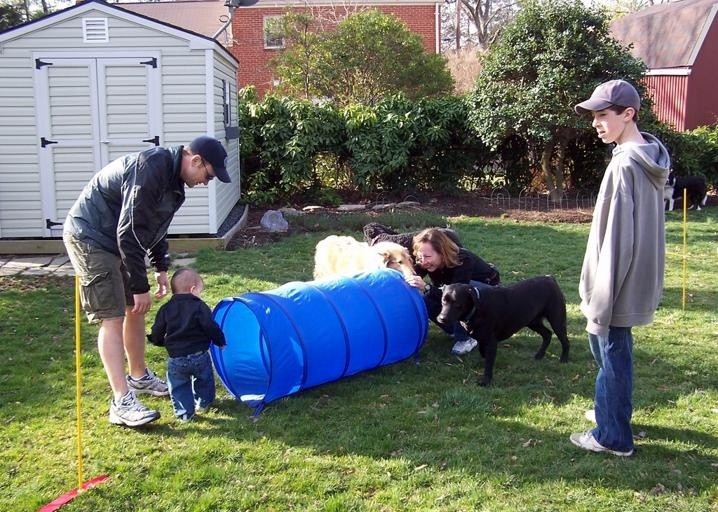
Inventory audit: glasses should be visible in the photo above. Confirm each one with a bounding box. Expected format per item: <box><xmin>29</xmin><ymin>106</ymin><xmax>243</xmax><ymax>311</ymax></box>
<box><xmin>201</xmin><ymin>159</ymin><xmax>214</xmax><ymax>181</ymax></box>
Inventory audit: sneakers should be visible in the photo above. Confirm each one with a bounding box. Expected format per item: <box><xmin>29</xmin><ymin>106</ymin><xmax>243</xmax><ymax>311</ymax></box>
<box><xmin>125</xmin><ymin>367</ymin><xmax>168</xmax><ymax>397</ymax></box>
<box><xmin>586</xmin><ymin>409</ymin><xmax>597</xmax><ymax>424</ymax></box>
<box><xmin>569</xmin><ymin>431</ymin><xmax>633</xmax><ymax>457</ymax></box>
<box><xmin>178</xmin><ymin>400</ymin><xmax>210</xmax><ymax>423</ymax></box>
<box><xmin>108</xmin><ymin>390</ymin><xmax>161</xmax><ymax>428</ymax></box>
<box><xmin>452</xmin><ymin>338</ymin><xmax>477</xmax><ymax>356</ymax></box>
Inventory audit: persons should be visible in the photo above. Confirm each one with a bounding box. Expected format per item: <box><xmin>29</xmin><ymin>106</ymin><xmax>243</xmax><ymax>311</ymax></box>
<box><xmin>61</xmin><ymin>136</ymin><xmax>231</xmax><ymax>428</ymax></box>
<box><xmin>412</xmin><ymin>229</ymin><xmax>501</xmax><ymax>356</ymax></box>
<box><xmin>146</xmin><ymin>267</ymin><xmax>226</xmax><ymax>422</ymax></box>
<box><xmin>568</xmin><ymin>80</ymin><xmax>670</xmax><ymax>459</ymax></box>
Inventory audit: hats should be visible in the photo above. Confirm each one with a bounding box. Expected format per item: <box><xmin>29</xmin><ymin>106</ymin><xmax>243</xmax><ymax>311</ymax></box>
<box><xmin>190</xmin><ymin>135</ymin><xmax>232</xmax><ymax>184</ymax></box>
<box><xmin>575</xmin><ymin>78</ymin><xmax>641</xmax><ymax>114</ymax></box>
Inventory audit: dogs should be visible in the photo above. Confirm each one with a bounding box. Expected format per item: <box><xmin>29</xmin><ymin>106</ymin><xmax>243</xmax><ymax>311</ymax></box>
<box><xmin>362</xmin><ymin>222</ymin><xmax>463</xmax><ymax>280</ymax></box>
<box><xmin>312</xmin><ymin>233</ymin><xmax>418</xmax><ymax>284</ymax></box>
<box><xmin>436</xmin><ymin>273</ymin><xmax>571</xmax><ymax>388</ymax></box>
